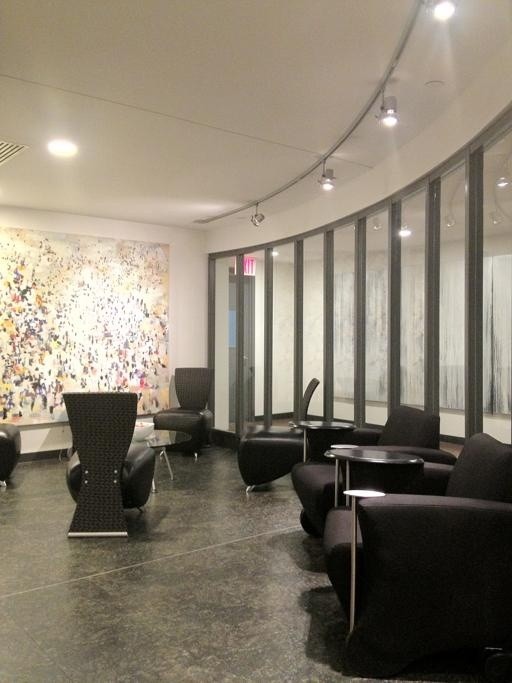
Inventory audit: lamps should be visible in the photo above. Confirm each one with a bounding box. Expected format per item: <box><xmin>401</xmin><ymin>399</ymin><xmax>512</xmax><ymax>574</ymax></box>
<box><xmin>425</xmin><ymin>1</ymin><xmax>456</xmax><ymax>20</ymax></box>
<box><xmin>380</xmin><ymin>88</ymin><xmax>398</xmax><ymax>127</ymax></box>
<box><xmin>399</xmin><ymin>224</ymin><xmax>411</xmax><ymax>237</ymax></box>
<box><xmin>444</xmin><ymin>212</ymin><xmax>456</xmax><ymax>227</ymax></box>
<box><xmin>489</xmin><ymin>211</ymin><xmax>503</xmax><ymax>225</ymax></box>
<box><xmin>272</xmin><ymin>249</ymin><xmax>280</xmax><ymax>258</ymax></box>
<box><xmin>322</xmin><ymin>160</ymin><xmax>334</xmax><ymax>191</ymax></box>
<box><xmin>371</xmin><ymin>218</ymin><xmax>383</xmax><ymax>231</ymax></box>
<box><xmin>251</xmin><ymin>204</ymin><xmax>265</xmax><ymax>226</ymax></box>
<box><xmin>496</xmin><ymin>176</ymin><xmax>511</xmax><ymax>189</ymax></box>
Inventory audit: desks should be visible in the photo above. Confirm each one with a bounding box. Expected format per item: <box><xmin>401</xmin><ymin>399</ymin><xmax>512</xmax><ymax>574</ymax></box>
<box><xmin>290</xmin><ymin>421</ymin><xmax>354</xmax><ymax>463</ymax></box>
<box><xmin>324</xmin><ymin>448</ymin><xmax>424</xmax><ymax>507</ymax></box>
<box><xmin>130</xmin><ymin>423</ymin><xmax>192</xmax><ymax>482</ymax></box>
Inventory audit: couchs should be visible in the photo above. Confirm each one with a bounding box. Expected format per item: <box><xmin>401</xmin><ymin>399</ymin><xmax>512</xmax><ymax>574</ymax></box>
<box><xmin>292</xmin><ymin>405</ymin><xmax>464</xmax><ymax>532</ymax></box>
<box><xmin>324</xmin><ymin>432</ymin><xmax>512</xmax><ymax>679</ymax></box>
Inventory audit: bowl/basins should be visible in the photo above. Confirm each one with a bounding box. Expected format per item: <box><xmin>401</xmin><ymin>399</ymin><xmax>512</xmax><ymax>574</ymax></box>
<box><xmin>132</xmin><ymin>422</ymin><xmax>155</xmax><ymax>441</ymax></box>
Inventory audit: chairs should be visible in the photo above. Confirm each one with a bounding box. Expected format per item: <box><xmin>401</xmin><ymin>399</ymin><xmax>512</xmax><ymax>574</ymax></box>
<box><xmin>153</xmin><ymin>367</ymin><xmax>215</xmax><ymax>460</ymax></box>
<box><xmin>238</xmin><ymin>379</ymin><xmax>318</xmax><ymax>493</ymax></box>
<box><xmin>0</xmin><ymin>423</ymin><xmax>20</xmax><ymax>487</ymax></box>
<box><xmin>63</xmin><ymin>392</ymin><xmax>156</xmax><ymax>537</ymax></box>
